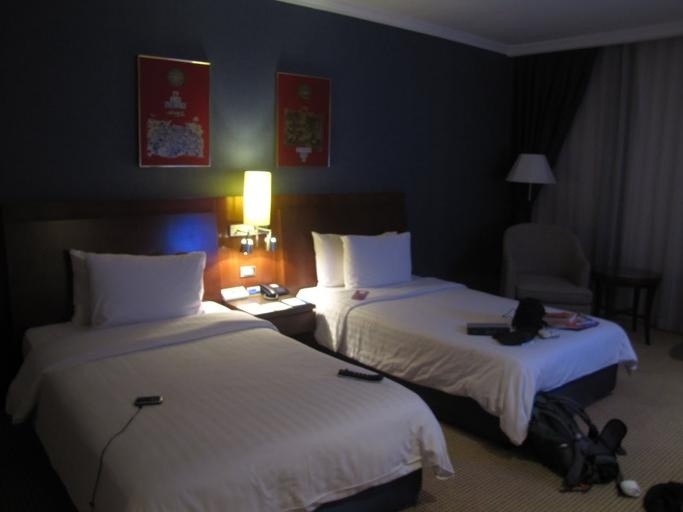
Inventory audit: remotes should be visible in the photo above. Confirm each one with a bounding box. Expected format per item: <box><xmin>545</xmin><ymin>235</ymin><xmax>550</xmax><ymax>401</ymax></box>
<box><xmin>337</xmin><ymin>366</ymin><xmax>384</xmax><ymax>383</ymax></box>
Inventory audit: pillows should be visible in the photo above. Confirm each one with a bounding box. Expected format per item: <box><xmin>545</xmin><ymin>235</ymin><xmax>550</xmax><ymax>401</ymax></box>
<box><xmin>68</xmin><ymin>248</ymin><xmax>92</xmax><ymax>326</ymax></box>
<box><xmin>85</xmin><ymin>251</ymin><xmax>207</xmax><ymax>329</ymax></box>
<box><xmin>339</xmin><ymin>231</ymin><xmax>412</xmax><ymax>289</ymax></box>
<box><xmin>311</xmin><ymin>230</ymin><xmax>398</xmax><ymax>288</ymax></box>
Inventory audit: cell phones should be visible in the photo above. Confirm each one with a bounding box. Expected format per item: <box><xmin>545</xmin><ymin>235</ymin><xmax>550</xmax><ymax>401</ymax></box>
<box><xmin>133</xmin><ymin>394</ymin><xmax>161</xmax><ymax>408</ymax></box>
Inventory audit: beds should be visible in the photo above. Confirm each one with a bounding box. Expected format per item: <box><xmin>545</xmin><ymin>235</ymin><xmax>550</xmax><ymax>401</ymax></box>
<box><xmin>1</xmin><ymin>197</ymin><xmax>456</xmax><ymax>512</ymax></box>
<box><xmin>277</xmin><ymin>193</ymin><xmax>639</xmax><ymax>449</ymax></box>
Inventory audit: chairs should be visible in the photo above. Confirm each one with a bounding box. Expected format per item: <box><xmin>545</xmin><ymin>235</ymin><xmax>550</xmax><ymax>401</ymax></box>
<box><xmin>500</xmin><ymin>222</ymin><xmax>594</xmax><ymax>315</ymax></box>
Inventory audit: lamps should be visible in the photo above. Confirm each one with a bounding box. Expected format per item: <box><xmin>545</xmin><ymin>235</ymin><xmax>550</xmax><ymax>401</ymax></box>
<box><xmin>229</xmin><ymin>170</ymin><xmax>277</xmax><ymax>255</ymax></box>
<box><xmin>504</xmin><ymin>153</ymin><xmax>557</xmax><ymax>202</ymax></box>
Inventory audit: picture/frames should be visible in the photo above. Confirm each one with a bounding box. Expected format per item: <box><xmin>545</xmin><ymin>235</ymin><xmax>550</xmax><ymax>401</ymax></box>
<box><xmin>137</xmin><ymin>54</ymin><xmax>211</xmax><ymax>169</ymax></box>
<box><xmin>276</xmin><ymin>71</ymin><xmax>332</xmax><ymax>169</ymax></box>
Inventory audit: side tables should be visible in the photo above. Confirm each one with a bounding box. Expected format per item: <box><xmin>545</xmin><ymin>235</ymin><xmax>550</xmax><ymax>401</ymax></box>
<box><xmin>596</xmin><ymin>264</ymin><xmax>662</xmax><ymax>345</ymax></box>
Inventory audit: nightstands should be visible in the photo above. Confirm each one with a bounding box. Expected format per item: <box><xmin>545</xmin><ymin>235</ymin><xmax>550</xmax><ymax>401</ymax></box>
<box><xmin>222</xmin><ymin>293</ymin><xmax>316</xmax><ymax>348</ymax></box>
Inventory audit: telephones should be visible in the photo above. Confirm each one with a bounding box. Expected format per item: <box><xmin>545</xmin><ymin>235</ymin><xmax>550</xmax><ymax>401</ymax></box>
<box><xmin>259</xmin><ymin>282</ymin><xmax>288</xmax><ymax>296</ymax></box>
